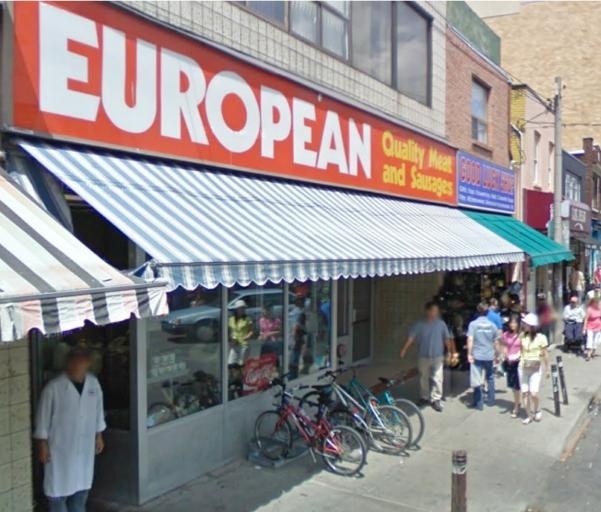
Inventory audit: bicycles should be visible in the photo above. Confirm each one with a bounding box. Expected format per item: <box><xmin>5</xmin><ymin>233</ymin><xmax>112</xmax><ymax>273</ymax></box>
<box><xmin>148</xmin><ymin>359</ymin><xmax>252</xmax><ymax>429</ymax></box>
<box><xmin>255</xmin><ymin>361</ymin><xmax>428</xmax><ymax>476</ymax></box>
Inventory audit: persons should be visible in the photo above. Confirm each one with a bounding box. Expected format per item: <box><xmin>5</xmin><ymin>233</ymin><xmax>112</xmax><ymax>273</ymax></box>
<box><xmin>399</xmin><ymin>264</ymin><xmax>601</xmax><ymax>425</ymax></box>
<box><xmin>227</xmin><ymin>291</ymin><xmax>331</xmax><ymax>382</ymax></box>
<box><xmin>32</xmin><ymin>346</ymin><xmax>108</xmax><ymax>512</ymax></box>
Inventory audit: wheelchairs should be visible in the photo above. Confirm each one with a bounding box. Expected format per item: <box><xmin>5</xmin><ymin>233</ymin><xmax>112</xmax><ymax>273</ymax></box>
<box><xmin>562</xmin><ymin>323</ymin><xmax>586</xmax><ymax>350</ymax></box>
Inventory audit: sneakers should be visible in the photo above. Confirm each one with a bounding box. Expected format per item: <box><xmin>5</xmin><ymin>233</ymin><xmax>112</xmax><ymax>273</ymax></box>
<box><xmin>433</xmin><ymin>400</ymin><xmax>443</xmax><ymax>411</ymax></box>
<box><xmin>416</xmin><ymin>398</ymin><xmax>429</xmax><ymax>408</ymax></box>
<box><xmin>536</xmin><ymin>410</ymin><xmax>543</xmax><ymax>421</ymax></box>
<box><xmin>511</xmin><ymin>408</ymin><xmax>520</xmax><ymax>418</ymax></box>
<box><xmin>523</xmin><ymin>414</ymin><xmax>534</xmax><ymax>424</ymax></box>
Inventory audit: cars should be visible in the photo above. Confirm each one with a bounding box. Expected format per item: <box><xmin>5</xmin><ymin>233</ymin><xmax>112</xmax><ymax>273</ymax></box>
<box><xmin>161</xmin><ymin>288</ymin><xmax>311</xmax><ymax>343</ymax></box>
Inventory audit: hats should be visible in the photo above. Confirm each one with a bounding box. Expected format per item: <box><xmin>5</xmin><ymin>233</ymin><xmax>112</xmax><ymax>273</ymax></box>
<box><xmin>570</xmin><ymin>296</ymin><xmax>578</xmax><ymax>303</ymax></box>
<box><xmin>523</xmin><ymin>313</ymin><xmax>540</xmax><ymax>327</ymax></box>
<box><xmin>228</xmin><ymin>299</ymin><xmax>249</xmax><ymax>310</ymax></box>
